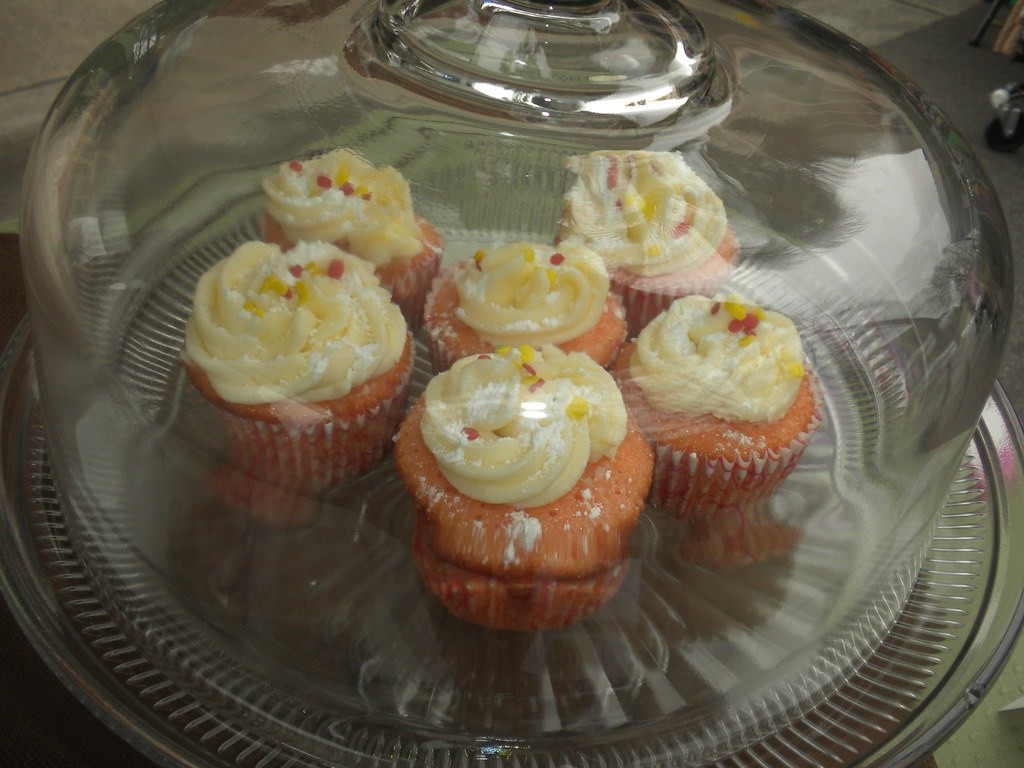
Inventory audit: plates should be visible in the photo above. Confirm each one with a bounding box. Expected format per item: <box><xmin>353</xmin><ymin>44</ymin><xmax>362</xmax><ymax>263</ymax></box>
<box><xmin>0</xmin><ymin>298</ymin><xmax>1024</xmax><ymax>768</ymax></box>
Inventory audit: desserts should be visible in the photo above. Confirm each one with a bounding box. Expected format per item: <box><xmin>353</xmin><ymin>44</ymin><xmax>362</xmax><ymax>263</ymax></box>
<box><xmin>179</xmin><ymin>148</ymin><xmax>824</xmax><ymax>631</ymax></box>
